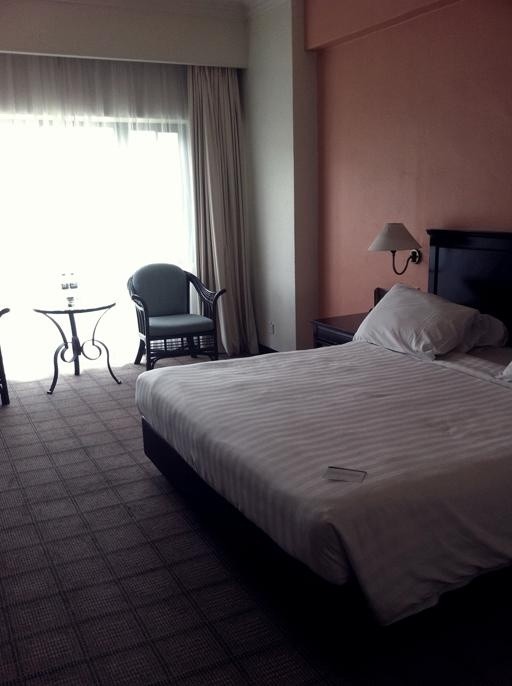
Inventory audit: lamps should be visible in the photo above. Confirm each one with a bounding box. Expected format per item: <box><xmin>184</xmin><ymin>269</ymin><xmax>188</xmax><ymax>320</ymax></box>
<box><xmin>367</xmin><ymin>222</ymin><xmax>423</xmax><ymax>275</ymax></box>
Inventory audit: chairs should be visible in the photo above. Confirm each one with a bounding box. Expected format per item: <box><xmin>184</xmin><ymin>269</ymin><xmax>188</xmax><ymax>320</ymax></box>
<box><xmin>127</xmin><ymin>264</ymin><xmax>227</xmax><ymax>370</ymax></box>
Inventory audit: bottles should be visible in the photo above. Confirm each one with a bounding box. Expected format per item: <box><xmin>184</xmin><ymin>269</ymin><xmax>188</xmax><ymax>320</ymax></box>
<box><xmin>61</xmin><ymin>272</ymin><xmax>78</xmax><ymax>303</ymax></box>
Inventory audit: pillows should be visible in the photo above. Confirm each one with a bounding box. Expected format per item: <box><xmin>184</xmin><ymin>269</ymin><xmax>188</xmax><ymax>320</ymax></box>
<box><xmin>352</xmin><ymin>283</ymin><xmax>512</xmax><ymax>381</ymax></box>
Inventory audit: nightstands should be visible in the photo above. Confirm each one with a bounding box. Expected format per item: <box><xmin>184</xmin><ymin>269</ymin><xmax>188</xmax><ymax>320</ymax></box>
<box><xmin>310</xmin><ymin>288</ymin><xmax>388</xmax><ymax>348</ymax></box>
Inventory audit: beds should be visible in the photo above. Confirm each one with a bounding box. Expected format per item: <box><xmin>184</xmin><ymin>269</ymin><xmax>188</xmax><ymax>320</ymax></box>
<box><xmin>142</xmin><ymin>228</ymin><xmax>512</xmax><ymax>676</ymax></box>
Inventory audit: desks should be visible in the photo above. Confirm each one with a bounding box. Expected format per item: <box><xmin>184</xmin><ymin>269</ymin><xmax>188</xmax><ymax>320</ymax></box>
<box><xmin>33</xmin><ymin>303</ymin><xmax>122</xmax><ymax>394</ymax></box>
